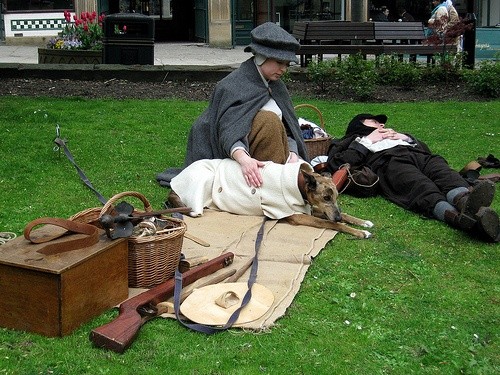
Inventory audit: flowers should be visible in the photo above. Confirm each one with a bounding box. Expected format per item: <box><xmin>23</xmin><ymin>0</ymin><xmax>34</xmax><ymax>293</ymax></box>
<box><xmin>48</xmin><ymin>10</ymin><xmax>126</xmax><ymax>49</ymax></box>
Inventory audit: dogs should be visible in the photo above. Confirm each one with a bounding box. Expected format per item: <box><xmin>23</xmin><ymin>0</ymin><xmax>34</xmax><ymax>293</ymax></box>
<box><xmin>166</xmin><ymin>164</ymin><xmax>375</xmax><ymax>240</ymax></box>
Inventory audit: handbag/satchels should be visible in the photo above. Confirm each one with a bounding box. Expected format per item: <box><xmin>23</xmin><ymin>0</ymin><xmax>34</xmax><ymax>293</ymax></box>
<box><xmin>339</xmin><ymin>163</ymin><xmax>379</xmax><ymax>198</ymax></box>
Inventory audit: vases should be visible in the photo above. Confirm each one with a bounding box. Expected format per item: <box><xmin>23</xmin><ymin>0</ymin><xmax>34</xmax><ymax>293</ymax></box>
<box><xmin>39</xmin><ymin>48</ymin><xmax>102</xmax><ymax>65</ymax></box>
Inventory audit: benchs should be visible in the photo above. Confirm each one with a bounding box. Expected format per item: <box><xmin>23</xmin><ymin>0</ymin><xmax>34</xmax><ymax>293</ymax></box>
<box><xmin>293</xmin><ymin>19</ymin><xmax>458</xmax><ymax>69</ymax></box>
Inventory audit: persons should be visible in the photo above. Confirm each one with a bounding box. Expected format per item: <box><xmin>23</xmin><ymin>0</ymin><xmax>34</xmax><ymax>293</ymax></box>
<box><xmin>423</xmin><ymin>0</ymin><xmax>463</xmax><ymax>46</ymax></box>
<box><xmin>185</xmin><ymin>22</ymin><xmax>311</xmax><ymax>188</ymax></box>
<box><xmin>371</xmin><ymin>7</ymin><xmax>416</xmax><ymax>22</ymax></box>
<box><xmin>328</xmin><ymin>114</ymin><xmax>500</xmax><ymax>242</ymax></box>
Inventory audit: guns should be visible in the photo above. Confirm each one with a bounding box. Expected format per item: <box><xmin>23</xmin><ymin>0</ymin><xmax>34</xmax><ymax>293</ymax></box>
<box><xmin>88</xmin><ymin>248</ymin><xmax>235</xmax><ymax>355</ymax></box>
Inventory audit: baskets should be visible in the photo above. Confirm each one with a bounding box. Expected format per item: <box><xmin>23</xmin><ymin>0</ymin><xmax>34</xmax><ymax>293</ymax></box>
<box><xmin>67</xmin><ymin>191</ymin><xmax>187</xmax><ymax>289</ymax></box>
<box><xmin>294</xmin><ymin>104</ymin><xmax>333</xmax><ymax>162</ymax></box>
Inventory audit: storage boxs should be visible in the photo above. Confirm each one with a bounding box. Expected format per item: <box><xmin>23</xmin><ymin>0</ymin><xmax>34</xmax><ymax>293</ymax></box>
<box><xmin>0</xmin><ymin>222</ymin><xmax>130</xmax><ymax>339</ymax></box>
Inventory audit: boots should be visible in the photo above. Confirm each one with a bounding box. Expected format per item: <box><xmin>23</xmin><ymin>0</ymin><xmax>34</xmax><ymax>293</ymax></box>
<box><xmin>444</xmin><ymin>206</ymin><xmax>500</xmax><ymax>243</ymax></box>
<box><xmin>453</xmin><ymin>181</ymin><xmax>496</xmax><ymax>229</ymax></box>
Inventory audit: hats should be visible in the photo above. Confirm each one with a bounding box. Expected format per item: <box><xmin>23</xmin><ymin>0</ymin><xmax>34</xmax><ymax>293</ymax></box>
<box><xmin>347</xmin><ymin>113</ymin><xmax>388</xmax><ymax>124</ymax></box>
<box><xmin>244</xmin><ymin>22</ymin><xmax>300</xmax><ymax>64</ymax></box>
